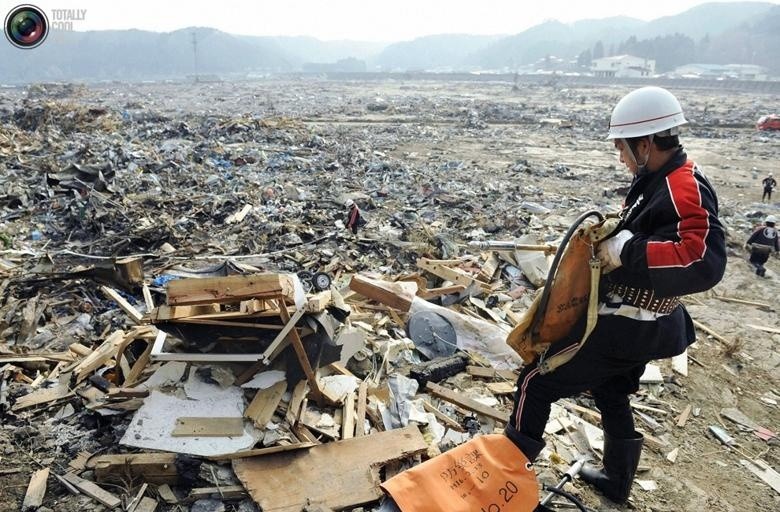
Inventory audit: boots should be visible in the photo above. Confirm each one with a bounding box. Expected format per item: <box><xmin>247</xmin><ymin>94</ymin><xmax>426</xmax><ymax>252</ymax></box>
<box><xmin>579</xmin><ymin>430</ymin><xmax>644</xmax><ymax>504</ymax></box>
<box><xmin>758</xmin><ymin>265</ymin><xmax>766</xmax><ymax>276</ymax></box>
<box><xmin>504</xmin><ymin>424</ymin><xmax>546</xmax><ymax>463</ymax></box>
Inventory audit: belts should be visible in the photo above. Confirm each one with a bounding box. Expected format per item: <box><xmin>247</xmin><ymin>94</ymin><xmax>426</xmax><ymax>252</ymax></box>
<box><xmin>614</xmin><ymin>284</ymin><xmax>679</xmax><ymax>314</ymax></box>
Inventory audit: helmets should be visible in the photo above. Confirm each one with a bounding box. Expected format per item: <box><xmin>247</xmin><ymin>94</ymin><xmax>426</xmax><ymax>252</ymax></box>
<box><xmin>765</xmin><ymin>215</ymin><xmax>776</xmax><ymax>224</ymax></box>
<box><xmin>604</xmin><ymin>85</ymin><xmax>688</xmax><ymax>141</ymax></box>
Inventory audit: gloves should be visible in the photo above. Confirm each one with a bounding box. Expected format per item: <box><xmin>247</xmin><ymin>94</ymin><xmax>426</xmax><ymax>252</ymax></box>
<box><xmin>596</xmin><ymin>230</ymin><xmax>632</xmax><ymax>276</ymax></box>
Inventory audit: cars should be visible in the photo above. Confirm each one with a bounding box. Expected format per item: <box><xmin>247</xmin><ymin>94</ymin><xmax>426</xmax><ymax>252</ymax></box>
<box><xmin>755</xmin><ymin>115</ymin><xmax>780</xmax><ymax>131</ymax></box>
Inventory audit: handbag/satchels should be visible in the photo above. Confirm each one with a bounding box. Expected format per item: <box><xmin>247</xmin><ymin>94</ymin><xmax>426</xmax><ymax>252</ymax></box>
<box><xmin>379</xmin><ymin>433</ymin><xmax>540</xmax><ymax>511</ymax></box>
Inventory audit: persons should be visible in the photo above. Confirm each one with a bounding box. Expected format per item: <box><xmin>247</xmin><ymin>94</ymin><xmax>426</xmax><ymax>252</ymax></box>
<box><xmin>744</xmin><ymin>214</ymin><xmax>780</xmax><ymax>277</ymax></box>
<box><xmin>503</xmin><ymin>85</ymin><xmax>728</xmax><ymax>505</ymax></box>
<box><xmin>762</xmin><ymin>173</ymin><xmax>777</xmax><ymax>203</ymax></box>
<box><xmin>345</xmin><ymin>199</ymin><xmax>361</xmax><ymax>236</ymax></box>
